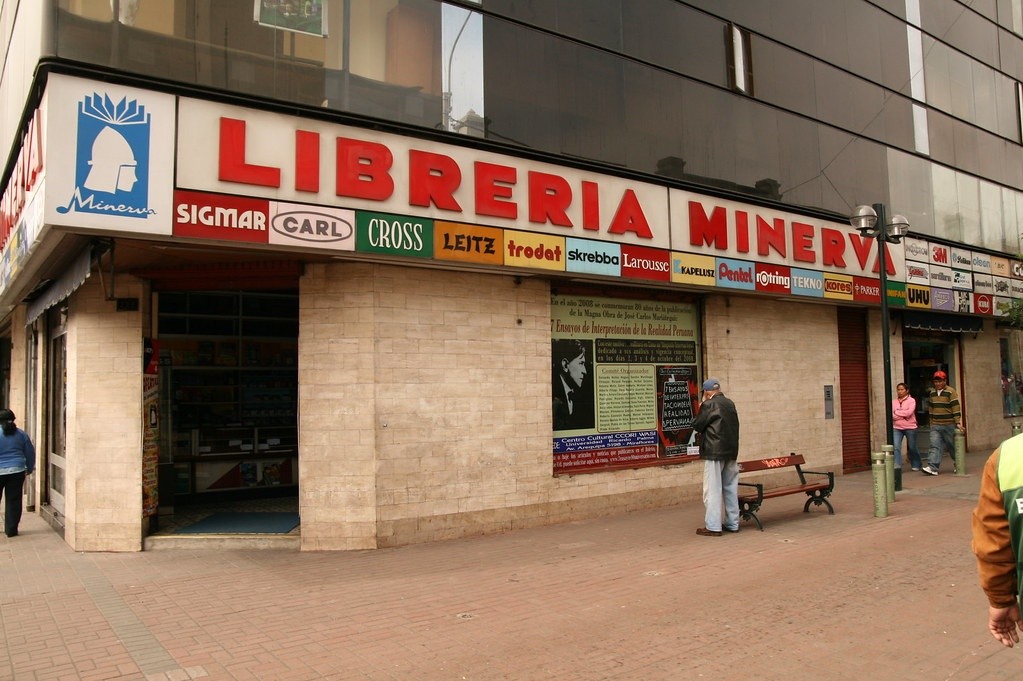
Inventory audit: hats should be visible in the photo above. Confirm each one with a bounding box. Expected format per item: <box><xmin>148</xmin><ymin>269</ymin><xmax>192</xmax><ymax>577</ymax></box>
<box><xmin>932</xmin><ymin>370</ymin><xmax>947</xmax><ymax>381</ymax></box>
<box><xmin>700</xmin><ymin>377</ymin><xmax>720</xmax><ymax>393</ymax></box>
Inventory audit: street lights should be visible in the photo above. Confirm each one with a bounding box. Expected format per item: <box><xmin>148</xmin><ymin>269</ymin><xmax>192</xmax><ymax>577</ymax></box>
<box><xmin>848</xmin><ymin>203</ymin><xmax>911</xmax><ymax>503</ymax></box>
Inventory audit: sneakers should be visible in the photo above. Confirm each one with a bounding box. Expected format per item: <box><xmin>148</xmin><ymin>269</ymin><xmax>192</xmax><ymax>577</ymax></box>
<box><xmin>923</xmin><ymin>466</ymin><xmax>939</xmax><ymax>476</ymax></box>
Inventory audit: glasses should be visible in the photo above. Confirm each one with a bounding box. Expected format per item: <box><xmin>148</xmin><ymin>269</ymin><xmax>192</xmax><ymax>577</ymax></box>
<box><xmin>933</xmin><ymin>377</ymin><xmax>942</xmax><ymax>380</ymax></box>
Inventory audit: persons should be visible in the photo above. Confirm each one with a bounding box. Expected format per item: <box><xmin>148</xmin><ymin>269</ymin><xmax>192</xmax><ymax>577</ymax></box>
<box><xmin>892</xmin><ymin>383</ymin><xmax>923</xmax><ymax>471</ymax></box>
<box><xmin>690</xmin><ymin>377</ymin><xmax>742</xmax><ymax>537</ymax></box>
<box><xmin>552</xmin><ymin>339</ymin><xmax>595</xmax><ymax>430</ymax></box>
<box><xmin>0</xmin><ymin>408</ymin><xmax>36</xmax><ymax>538</ymax></box>
<box><xmin>1000</xmin><ymin>371</ymin><xmax>1023</xmax><ymax>417</ymax></box>
<box><xmin>922</xmin><ymin>371</ymin><xmax>966</xmax><ymax>475</ymax></box>
<box><xmin>971</xmin><ymin>430</ymin><xmax>1023</xmax><ymax>648</ymax></box>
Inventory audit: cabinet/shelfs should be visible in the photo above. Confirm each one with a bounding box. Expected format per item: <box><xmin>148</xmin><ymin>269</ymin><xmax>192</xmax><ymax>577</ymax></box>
<box><xmin>151</xmin><ymin>291</ymin><xmax>299</xmax><ymax>457</ymax></box>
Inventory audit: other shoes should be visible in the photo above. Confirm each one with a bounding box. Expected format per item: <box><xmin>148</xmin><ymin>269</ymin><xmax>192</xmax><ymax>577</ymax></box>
<box><xmin>911</xmin><ymin>467</ymin><xmax>922</xmax><ymax>472</ymax></box>
<box><xmin>722</xmin><ymin>524</ymin><xmax>739</xmax><ymax>533</ymax></box>
<box><xmin>4</xmin><ymin>530</ymin><xmax>19</xmax><ymax>538</ymax></box>
<box><xmin>696</xmin><ymin>528</ymin><xmax>723</xmax><ymax>536</ymax></box>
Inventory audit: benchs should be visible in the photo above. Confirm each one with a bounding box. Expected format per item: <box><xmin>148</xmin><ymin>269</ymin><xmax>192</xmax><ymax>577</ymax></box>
<box><xmin>735</xmin><ymin>452</ymin><xmax>834</xmax><ymax>532</ymax></box>
